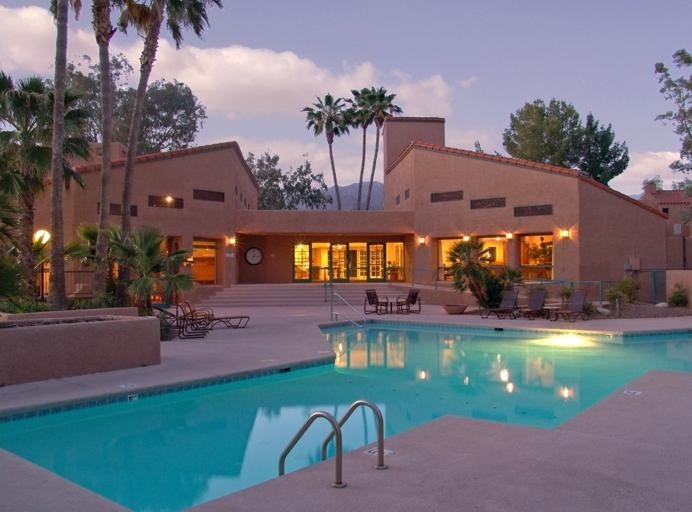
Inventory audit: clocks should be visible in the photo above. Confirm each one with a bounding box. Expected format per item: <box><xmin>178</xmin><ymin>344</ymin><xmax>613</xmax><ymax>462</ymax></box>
<box><xmin>245</xmin><ymin>247</ymin><xmax>264</xmax><ymax>266</ymax></box>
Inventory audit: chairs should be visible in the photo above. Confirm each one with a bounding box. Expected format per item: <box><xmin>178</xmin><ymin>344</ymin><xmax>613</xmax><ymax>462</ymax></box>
<box><xmin>151</xmin><ymin>299</ymin><xmax>250</xmax><ymax>339</ymax></box>
<box><xmin>363</xmin><ymin>288</ymin><xmax>421</xmax><ymax>316</ymax></box>
<box><xmin>479</xmin><ymin>288</ymin><xmax>591</xmax><ymax>322</ymax></box>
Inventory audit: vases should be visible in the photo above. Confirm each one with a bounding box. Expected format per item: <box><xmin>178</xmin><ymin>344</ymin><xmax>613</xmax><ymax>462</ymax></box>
<box><xmin>443</xmin><ymin>304</ymin><xmax>468</xmax><ymax>314</ymax></box>
